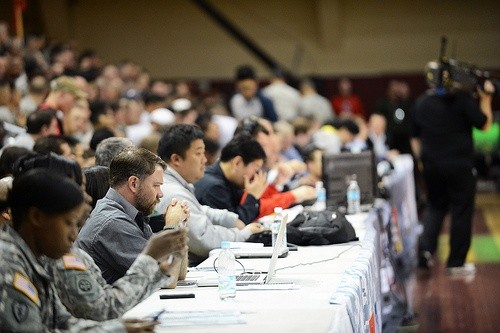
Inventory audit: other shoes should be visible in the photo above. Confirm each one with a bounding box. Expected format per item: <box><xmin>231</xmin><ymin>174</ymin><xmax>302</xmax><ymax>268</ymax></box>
<box><xmin>447</xmin><ymin>263</ymin><xmax>475</xmax><ymax>276</ymax></box>
<box><xmin>416</xmin><ymin>250</ymin><xmax>434</xmax><ymax>268</ymax></box>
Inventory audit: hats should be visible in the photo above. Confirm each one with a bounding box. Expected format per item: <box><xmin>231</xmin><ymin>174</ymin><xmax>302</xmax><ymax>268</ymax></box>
<box><xmin>50</xmin><ymin>75</ymin><xmax>90</xmax><ymax>100</ymax></box>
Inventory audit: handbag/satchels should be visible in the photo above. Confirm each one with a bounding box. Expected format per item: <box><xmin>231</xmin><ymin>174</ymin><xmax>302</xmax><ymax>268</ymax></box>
<box><xmin>245</xmin><ymin>210</ymin><xmax>356</xmax><ymax>245</ymax></box>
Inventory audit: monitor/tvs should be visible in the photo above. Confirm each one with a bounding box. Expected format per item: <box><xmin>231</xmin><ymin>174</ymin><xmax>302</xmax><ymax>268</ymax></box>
<box><xmin>321</xmin><ymin>149</ymin><xmax>374</xmax><ymax>210</ymax></box>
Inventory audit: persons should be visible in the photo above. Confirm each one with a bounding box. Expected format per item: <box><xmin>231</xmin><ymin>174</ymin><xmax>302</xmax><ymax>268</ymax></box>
<box><xmin>404</xmin><ymin>62</ymin><xmax>493</xmax><ymax>275</ymax></box>
<box><xmin>0</xmin><ymin>0</ymin><xmax>421</xmax><ymax>333</ymax></box>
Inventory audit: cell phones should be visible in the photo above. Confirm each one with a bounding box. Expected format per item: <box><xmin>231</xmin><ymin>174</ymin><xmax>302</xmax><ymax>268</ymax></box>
<box><xmin>160</xmin><ymin>293</ymin><xmax>195</xmax><ymax>300</ymax></box>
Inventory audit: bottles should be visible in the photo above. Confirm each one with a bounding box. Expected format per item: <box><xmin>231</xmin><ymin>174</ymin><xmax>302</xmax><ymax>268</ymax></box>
<box><xmin>315</xmin><ymin>181</ymin><xmax>326</xmax><ymax>210</ymax></box>
<box><xmin>347</xmin><ymin>181</ymin><xmax>360</xmax><ymax>215</ymax></box>
<box><xmin>217</xmin><ymin>242</ymin><xmax>236</xmax><ymax>301</ymax></box>
<box><xmin>271</xmin><ymin>207</ymin><xmax>286</xmax><ymax>254</ymax></box>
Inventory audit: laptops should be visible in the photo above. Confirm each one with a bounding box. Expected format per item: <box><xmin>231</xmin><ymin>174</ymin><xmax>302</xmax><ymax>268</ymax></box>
<box><xmin>195</xmin><ymin>211</ymin><xmax>289</xmax><ymax>286</ymax></box>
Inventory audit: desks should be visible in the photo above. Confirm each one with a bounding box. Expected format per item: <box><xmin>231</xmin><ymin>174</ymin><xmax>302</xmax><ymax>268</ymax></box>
<box><xmin>116</xmin><ymin>155</ymin><xmax>417</xmax><ymax>333</ymax></box>
